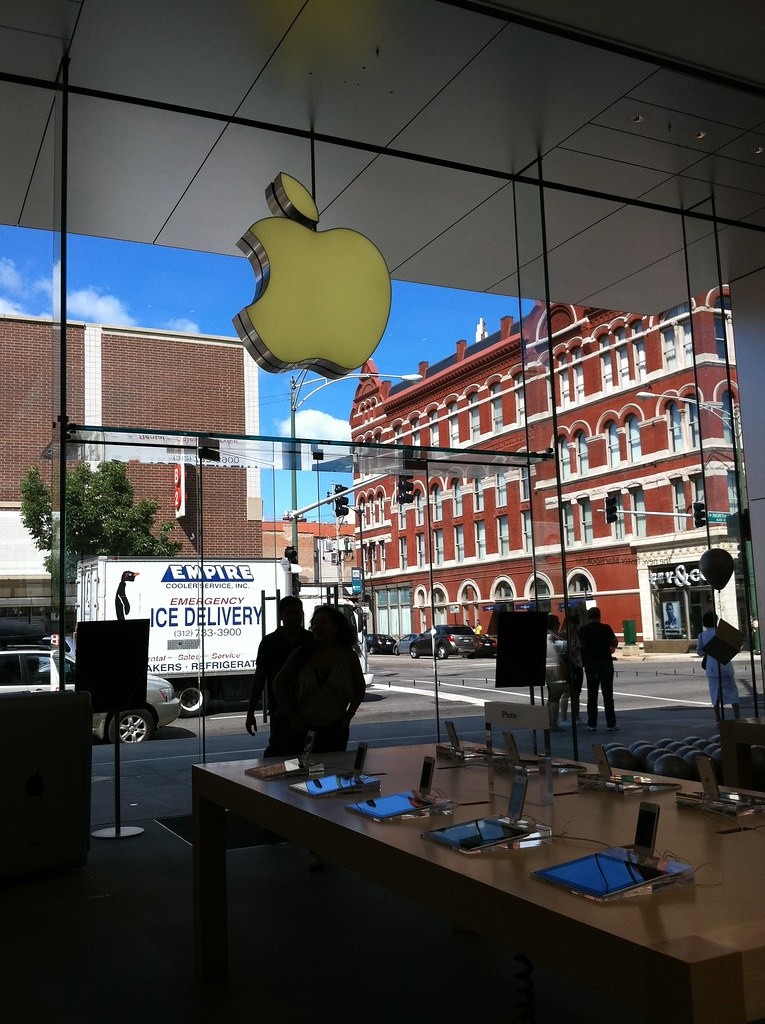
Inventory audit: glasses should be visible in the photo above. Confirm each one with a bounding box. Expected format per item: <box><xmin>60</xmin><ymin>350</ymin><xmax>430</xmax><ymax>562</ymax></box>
<box><xmin>284</xmin><ymin>609</ymin><xmax>301</xmax><ymax>616</ymax></box>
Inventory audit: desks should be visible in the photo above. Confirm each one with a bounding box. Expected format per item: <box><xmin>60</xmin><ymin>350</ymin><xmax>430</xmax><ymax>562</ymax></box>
<box><xmin>721</xmin><ymin>716</ymin><xmax>765</xmax><ymax>785</ymax></box>
<box><xmin>0</xmin><ymin>689</ymin><xmax>92</xmax><ymax>879</ymax></box>
<box><xmin>188</xmin><ymin>735</ymin><xmax>765</xmax><ymax>1024</ymax></box>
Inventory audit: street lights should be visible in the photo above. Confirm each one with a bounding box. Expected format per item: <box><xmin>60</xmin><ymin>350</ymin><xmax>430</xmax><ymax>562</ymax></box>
<box><xmin>326</xmin><ymin>490</ymin><xmax>365</xmax><ymax>606</ymax></box>
<box><xmin>636</xmin><ymin>391</ymin><xmax>761</xmax><ymax>652</ymax></box>
<box><xmin>289</xmin><ymin>373</ymin><xmax>423</xmax><ymax>557</ymax></box>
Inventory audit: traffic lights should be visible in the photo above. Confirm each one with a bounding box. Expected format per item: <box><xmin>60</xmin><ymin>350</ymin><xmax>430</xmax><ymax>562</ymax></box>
<box><xmin>398</xmin><ymin>475</ymin><xmax>414</xmax><ymax>504</ymax></box>
<box><xmin>604</xmin><ymin>497</ymin><xmax>618</xmax><ymax>523</ymax></box>
<box><xmin>692</xmin><ymin>501</ymin><xmax>707</xmax><ymax>528</ymax></box>
<box><xmin>335</xmin><ymin>485</ymin><xmax>349</xmax><ymax>516</ymax></box>
<box><xmin>285</xmin><ymin>548</ymin><xmax>298</xmax><ymax>565</ymax></box>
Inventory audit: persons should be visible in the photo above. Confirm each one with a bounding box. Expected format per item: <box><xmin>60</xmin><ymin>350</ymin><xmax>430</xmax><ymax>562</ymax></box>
<box><xmin>664</xmin><ymin>603</ymin><xmax>676</xmax><ymax>627</ymax></box>
<box><xmin>578</xmin><ymin>607</ymin><xmax>620</xmax><ymax>732</ymax></box>
<box><xmin>557</xmin><ymin>613</ymin><xmax>588</xmax><ymax>728</ymax></box>
<box><xmin>271</xmin><ymin>605</ymin><xmax>369</xmax><ymax>753</ymax></box>
<box><xmin>245</xmin><ymin>596</ymin><xmax>315</xmax><ymax>758</ymax></box>
<box><xmin>544</xmin><ymin>615</ymin><xmax>567</xmax><ymax>732</ymax></box>
<box><xmin>696</xmin><ymin>611</ymin><xmax>740</xmax><ymax>729</ymax></box>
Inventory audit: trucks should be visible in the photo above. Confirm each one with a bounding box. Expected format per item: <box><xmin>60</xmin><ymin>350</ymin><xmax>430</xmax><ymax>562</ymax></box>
<box><xmin>75</xmin><ymin>555</ymin><xmax>376</xmax><ymax>718</ymax></box>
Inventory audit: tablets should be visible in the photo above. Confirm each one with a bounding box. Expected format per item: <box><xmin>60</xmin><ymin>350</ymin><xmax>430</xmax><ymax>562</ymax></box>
<box><xmin>246</xmin><ymin>744</ymin><xmax>765</xmax><ymax>898</ymax></box>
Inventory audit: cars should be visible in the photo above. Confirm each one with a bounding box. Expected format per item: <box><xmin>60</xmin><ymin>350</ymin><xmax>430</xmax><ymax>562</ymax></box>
<box><xmin>467</xmin><ymin>634</ymin><xmax>497</xmax><ymax>659</ymax></box>
<box><xmin>409</xmin><ymin>623</ymin><xmax>477</xmax><ymax>660</ymax></box>
<box><xmin>0</xmin><ymin>617</ymin><xmax>182</xmax><ymax>744</ymax></box>
<box><xmin>393</xmin><ymin>633</ymin><xmax>423</xmax><ymax>656</ymax></box>
<box><xmin>366</xmin><ymin>634</ymin><xmax>397</xmax><ymax>655</ymax></box>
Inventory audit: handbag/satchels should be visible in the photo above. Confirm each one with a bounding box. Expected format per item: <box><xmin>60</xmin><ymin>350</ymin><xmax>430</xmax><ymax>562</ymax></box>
<box><xmin>701</xmin><ymin>655</ymin><xmax>707</xmax><ymax>669</ymax></box>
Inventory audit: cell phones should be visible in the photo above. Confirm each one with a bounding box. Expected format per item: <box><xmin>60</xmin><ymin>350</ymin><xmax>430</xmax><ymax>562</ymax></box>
<box><xmin>508</xmin><ymin>775</ymin><xmax>528</xmax><ymax>820</ymax></box>
<box><xmin>502</xmin><ymin>732</ymin><xmax>520</xmax><ymax>760</ymax></box>
<box><xmin>354</xmin><ymin>742</ymin><xmax>368</xmax><ymax>775</ymax></box>
<box><xmin>633</xmin><ymin>801</ymin><xmax>660</xmax><ymax>858</ymax></box>
<box><xmin>418</xmin><ymin>756</ymin><xmax>435</xmax><ymax>795</ymax></box>
<box><xmin>444</xmin><ymin>720</ymin><xmax>459</xmax><ymax>747</ymax></box>
<box><xmin>304</xmin><ymin>731</ymin><xmax>315</xmax><ymax>754</ymax></box>
<box><xmin>591</xmin><ymin>743</ymin><xmax>612</xmax><ymax>776</ymax></box>
<box><xmin>695</xmin><ymin>757</ymin><xmax>720</xmax><ymax>797</ymax></box>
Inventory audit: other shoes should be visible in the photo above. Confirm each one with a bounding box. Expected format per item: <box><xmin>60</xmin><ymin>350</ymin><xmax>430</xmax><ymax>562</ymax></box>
<box><xmin>576</xmin><ymin>717</ymin><xmax>588</xmax><ymax>726</ymax></box>
<box><xmin>550</xmin><ymin>725</ymin><xmax>564</xmax><ymax>732</ymax></box>
<box><xmin>309</xmin><ymin>853</ymin><xmax>325</xmax><ymax>870</ymax></box>
<box><xmin>606</xmin><ymin>724</ymin><xmax>619</xmax><ymax>731</ymax></box>
<box><xmin>559</xmin><ymin>720</ymin><xmax>574</xmax><ymax>729</ymax></box>
<box><xmin>586</xmin><ymin>725</ymin><xmax>598</xmax><ymax>730</ymax></box>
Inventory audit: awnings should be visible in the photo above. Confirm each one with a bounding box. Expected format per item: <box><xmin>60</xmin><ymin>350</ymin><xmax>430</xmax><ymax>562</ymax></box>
<box><xmin>483</xmin><ymin>604</ymin><xmax>510</xmax><ymax>610</ymax></box>
<box><xmin>515</xmin><ymin>600</ymin><xmax>549</xmax><ymax>609</ymax></box>
<box><xmin>561</xmin><ymin>598</ymin><xmax>594</xmax><ymax>608</ymax></box>
